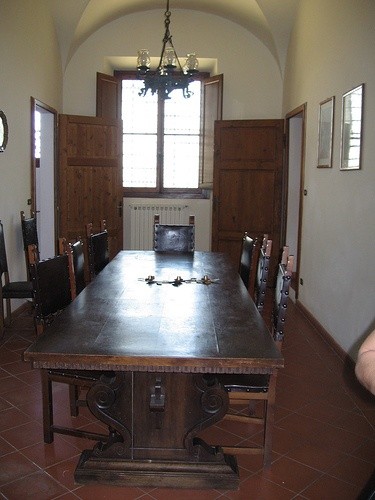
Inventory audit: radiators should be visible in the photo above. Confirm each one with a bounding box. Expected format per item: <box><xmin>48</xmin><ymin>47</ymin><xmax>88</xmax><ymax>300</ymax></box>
<box><xmin>128</xmin><ymin>202</ymin><xmax>191</xmax><ymax>251</ymax></box>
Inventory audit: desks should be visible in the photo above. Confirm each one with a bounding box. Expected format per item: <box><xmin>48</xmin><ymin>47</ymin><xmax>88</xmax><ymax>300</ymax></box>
<box><xmin>22</xmin><ymin>249</ymin><xmax>286</xmax><ymax>487</ymax></box>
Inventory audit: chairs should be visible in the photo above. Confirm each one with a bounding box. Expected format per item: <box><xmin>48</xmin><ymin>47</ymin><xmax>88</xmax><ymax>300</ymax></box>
<box><xmin>182</xmin><ymin>228</ymin><xmax>296</xmax><ymax>471</ymax></box>
<box><xmin>0</xmin><ymin>209</ymin><xmax>113</xmax><ymax>444</ymax></box>
<box><xmin>151</xmin><ymin>212</ymin><xmax>196</xmax><ymax>255</ymax></box>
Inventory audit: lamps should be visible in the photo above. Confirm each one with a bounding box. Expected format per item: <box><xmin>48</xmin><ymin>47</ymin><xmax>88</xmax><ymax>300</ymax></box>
<box><xmin>133</xmin><ymin>0</ymin><xmax>200</xmax><ymax>101</ymax></box>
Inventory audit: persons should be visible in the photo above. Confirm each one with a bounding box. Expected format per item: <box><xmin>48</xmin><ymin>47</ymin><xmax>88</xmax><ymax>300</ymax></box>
<box><xmin>355</xmin><ymin>330</ymin><xmax>375</xmax><ymax>500</ymax></box>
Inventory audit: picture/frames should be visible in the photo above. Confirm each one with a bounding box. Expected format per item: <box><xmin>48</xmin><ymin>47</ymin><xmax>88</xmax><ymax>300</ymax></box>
<box><xmin>338</xmin><ymin>82</ymin><xmax>365</xmax><ymax>172</ymax></box>
<box><xmin>315</xmin><ymin>96</ymin><xmax>335</xmax><ymax>169</ymax></box>
